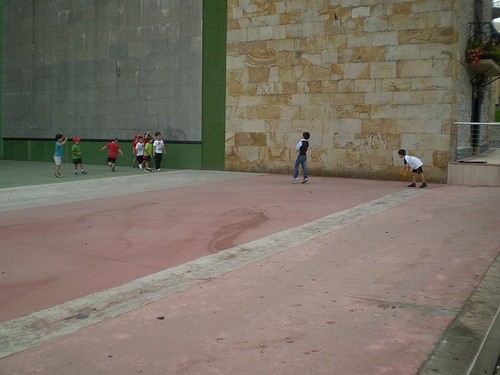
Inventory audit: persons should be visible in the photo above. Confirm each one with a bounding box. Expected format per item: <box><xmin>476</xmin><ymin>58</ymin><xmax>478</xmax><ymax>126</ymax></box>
<box><xmin>398</xmin><ymin>148</ymin><xmax>427</xmax><ymax>188</ymax></box>
<box><xmin>153</xmin><ymin>131</ymin><xmax>165</xmax><ymax>171</ymax></box>
<box><xmin>132</xmin><ymin>128</ymin><xmax>153</xmax><ymax>173</ymax></box>
<box><xmin>71</xmin><ymin>136</ymin><xmax>88</xmax><ymax>175</ymax></box>
<box><xmin>289</xmin><ymin>131</ymin><xmax>310</xmax><ymax>183</ymax></box>
<box><xmin>53</xmin><ymin>133</ymin><xmax>68</xmax><ymax>178</ymax></box>
<box><xmin>100</xmin><ymin>137</ymin><xmax>123</xmax><ymax>172</ymax></box>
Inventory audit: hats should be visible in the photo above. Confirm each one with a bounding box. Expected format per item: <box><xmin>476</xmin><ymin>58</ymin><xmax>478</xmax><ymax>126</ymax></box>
<box><xmin>73</xmin><ymin>136</ymin><xmax>81</xmax><ymax>142</ymax></box>
<box><xmin>135</xmin><ymin>134</ymin><xmax>140</xmax><ymax>138</ymax></box>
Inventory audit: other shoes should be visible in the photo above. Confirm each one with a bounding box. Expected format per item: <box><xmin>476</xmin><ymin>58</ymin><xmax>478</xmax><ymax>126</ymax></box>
<box><xmin>82</xmin><ymin>171</ymin><xmax>88</xmax><ymax>174</ymax></box>
<box><xmin>55</xmin><ymin>173</ymin><xmax>63</xmax><ymax>178</ymax></box>
<box><xmin>75</xmin><ymin>171</ymin><xmax>78</xmax><ymax>175</ymax></box>
<box><xmin>139</xmin><ymin>163</ymin><xmax>144</xmax><ymax>169</ymax></box>
<box><xmin>302</xmin><ymin>177</ymin><xmax>308</xmax><ymax>184</ymax></box>
<box><xmin>291</xmin><ymin>176</ymin><xmax>299</xmax><ymax>182</ymax></box>
<box><xmin>419</xmin><ymin>183</ymin><xmax>427</xmax><ymax>188</ymax></box>
<box><xmin>408</xmin><ymin>183</ymin><xmax>416</xmax><ymax>187</ymax></box>
